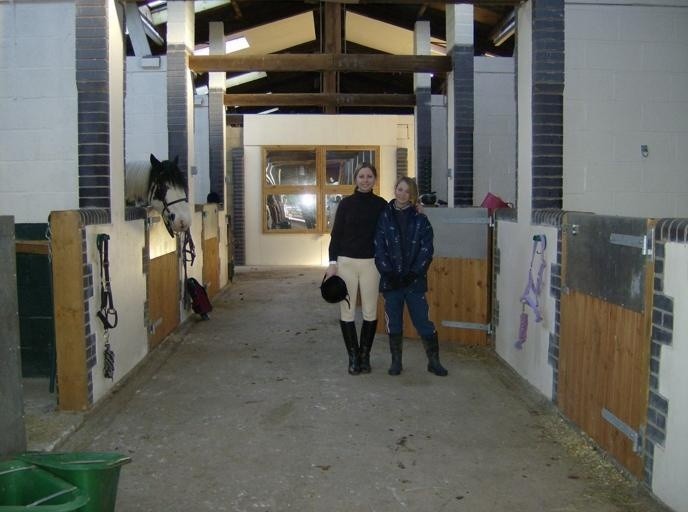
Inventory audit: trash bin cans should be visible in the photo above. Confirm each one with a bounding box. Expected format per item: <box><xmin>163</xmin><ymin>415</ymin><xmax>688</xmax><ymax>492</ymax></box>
<box><xmin>0</xmin><ymin>460</ymin><xmax>91</xmax><ymax>512</ymax></box>
<box><xmin>6</xmin><ymin>450</ymin><xmax>134</xmax><ymax>512</ymax></box>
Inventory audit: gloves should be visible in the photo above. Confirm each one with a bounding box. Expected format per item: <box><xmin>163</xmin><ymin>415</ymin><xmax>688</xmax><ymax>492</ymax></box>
<box><xmin>389</xmin><ymin>278</ymin><xmax>399</xmax><ymax>290</ymax></box>
<box><xmin>400</xmin><ymin>271</ymin><xmax>418</xmax><ymax>287</ymax></box>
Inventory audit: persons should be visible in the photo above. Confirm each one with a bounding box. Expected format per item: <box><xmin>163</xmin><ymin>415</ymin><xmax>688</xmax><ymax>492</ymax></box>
<box><xmin>373</xmin><ymin>175</ymin><xmax>449</xmax><ymax>379</ymax></box>
<box><xmin>323</xmin><ymin>160</ymin><xmax>424</xmax><ymax>376</ymax></box>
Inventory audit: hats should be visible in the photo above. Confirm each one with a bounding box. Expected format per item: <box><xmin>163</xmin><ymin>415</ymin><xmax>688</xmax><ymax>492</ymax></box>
<box><xmin>419</xmin><ymin>191</ymin><xmax>436</xmax><ymax>204</ymax></box>
<box><xmin>320</xmin><ymin>275</ymin><xmax>347</xmax><ymax>303</ymax></box>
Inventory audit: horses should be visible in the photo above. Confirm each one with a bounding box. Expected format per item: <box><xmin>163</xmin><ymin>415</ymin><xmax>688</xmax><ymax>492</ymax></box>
<box><xmin>124</xmin><ymin>153</ymin><xmax>192</xmax><ymax>232</ymax></box>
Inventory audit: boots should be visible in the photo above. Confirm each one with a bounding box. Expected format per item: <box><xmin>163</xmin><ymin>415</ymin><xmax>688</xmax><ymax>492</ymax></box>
<box><xmin>340</xmin><ymin>319</ymin><xmax>360</xmax><ymax>374</ymax></box>
<box><xmin>389</xmin><ymin>335</ymin><xmax>402</xmax><ymax>375</ymax></box>
<box><xmin>360</xmin><ymin>319</ymin><xmax>377</xmax><ymax>373</ymax></box>
<box><xmin>422</xmin><ymin>332</ymin><xmax>448</xmax><ymax>376</ymax></box>
